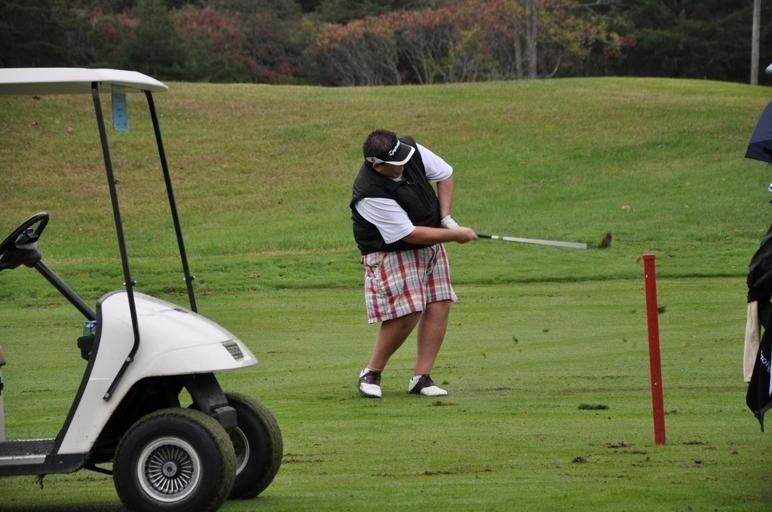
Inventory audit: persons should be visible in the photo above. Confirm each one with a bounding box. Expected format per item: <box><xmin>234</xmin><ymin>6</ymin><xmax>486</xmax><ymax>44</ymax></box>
<box><xmin>349</xmin><ymin>128</ymin><xmax>479</xmax><ymax>400</ymax></box>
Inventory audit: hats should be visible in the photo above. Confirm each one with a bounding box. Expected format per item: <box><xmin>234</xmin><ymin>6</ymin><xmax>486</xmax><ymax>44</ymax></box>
<box><xmin>365</xmin><ymin>136</ymin><xmax>416</xmax><ymax>166</ymax></box>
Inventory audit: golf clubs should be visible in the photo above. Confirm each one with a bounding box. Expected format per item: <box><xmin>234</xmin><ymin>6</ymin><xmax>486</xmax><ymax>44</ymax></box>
<box><xmin>476</xmin><ymin>232</ymin><xmax>612</xmax><ymax>249</ymax></box>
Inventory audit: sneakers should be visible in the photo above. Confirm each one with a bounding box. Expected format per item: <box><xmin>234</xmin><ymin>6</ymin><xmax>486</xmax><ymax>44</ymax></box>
<box><xmin>408</xmin><ymin>374</ymin><xmax>447</xmax><ymax>397</ymax></box>
<box><xmin>359</xmin><ymin>369</ymin><xmax>382</xmax><ymax>399</ymax></box>
